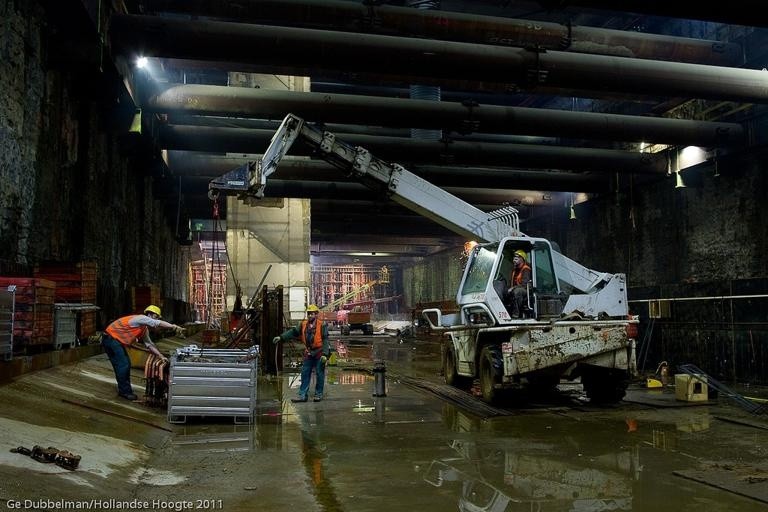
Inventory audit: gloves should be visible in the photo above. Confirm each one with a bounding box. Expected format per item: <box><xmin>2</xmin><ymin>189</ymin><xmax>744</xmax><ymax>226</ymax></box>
<box><xmin>167</xmin><ymin>324</ymin><xmax>188</xmax><ymax>341</ymax></box>
<box><xmin>320</xmin><ymin>355</ymin><xmax>327</xmax><ymax>365</ymax></box>
<box><xmin>159</xmin><ymin>354</ymin><xmax>170</xmax><ymax>365</ymax></box>
<box><xmin>273</xmin><ymin>336</ymin><xmax>282</xmax><ymax>344</ymax></box>
<box><xmin>507</xmin><ymin>286</ymin><xmax>514</xmax><ymax>294</ymax></box>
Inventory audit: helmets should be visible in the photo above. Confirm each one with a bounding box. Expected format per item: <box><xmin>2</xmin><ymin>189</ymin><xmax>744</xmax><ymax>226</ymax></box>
<box><xmin>306</xmin><ymin>304</ymin><xmax>318</xmax><ymax>313</ymax></box>
<box><xmin>144</xmin><ymin>304</ymin><xmax>162</xmax><ymax>319</ymax></box>
<box><xmin>514</xmin><ymin>249</ymin><xmax>527</xmax><ymax>262</ymax></box>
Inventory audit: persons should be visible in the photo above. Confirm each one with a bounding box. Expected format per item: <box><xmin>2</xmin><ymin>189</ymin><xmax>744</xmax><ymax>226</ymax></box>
<box><xmin>102</xmin><ymin>304</ymin><xmax>188</xmax><ymax>400</ymax></box>
<box><xmin>506</xmin><ymin>250</ymin><xmax>532</xmax><ymax>320</ymax></box>
<box><xmin>273</xmin><ymin>304</ymin><xmax>333</xmax><ymax>404</ymax></box>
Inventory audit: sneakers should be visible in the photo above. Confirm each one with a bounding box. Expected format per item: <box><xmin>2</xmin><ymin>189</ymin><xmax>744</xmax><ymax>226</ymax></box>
<box><xmin>313</xmin><ymin>396</ymin><xmax>322</xmax><ymax>403</ymax></box>
<box><xmin>290</xmin><ymin>396</ymin><xmax>308</xmax><ymax>403</ymax></box>
<box><xmin>511</xmin><ymin>313</ymin><xmax>519</xmax><ymax>318</ymax></box>
<box><xmin>119</xmin><ymin>391</ymin><xmax>138</xmax><ymax>401</ymax></box>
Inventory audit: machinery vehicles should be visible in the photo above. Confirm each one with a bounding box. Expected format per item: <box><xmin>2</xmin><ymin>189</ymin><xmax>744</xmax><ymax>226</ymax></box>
<box><xmin>200</xmin><ymin>110</ymin><xmax>639</xmax><ymax>407</ymax></box>
<box><xmin>334</xmin><ymin>298</ymin><xmax>380</xmax><ymax>336</ymax></box>
